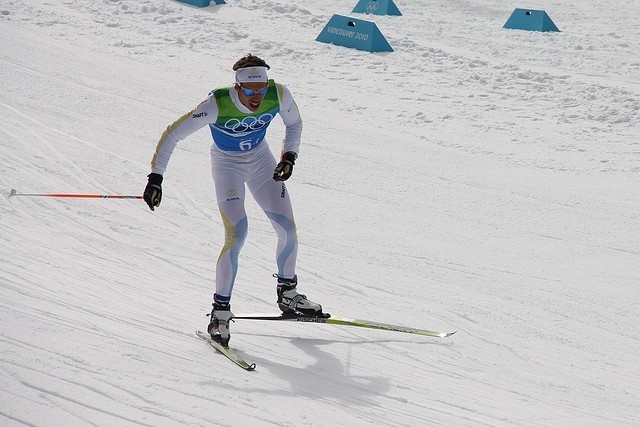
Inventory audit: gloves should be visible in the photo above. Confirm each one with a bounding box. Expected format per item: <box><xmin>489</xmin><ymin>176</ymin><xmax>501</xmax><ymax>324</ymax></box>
<box><xmin>143</xmin><ymin>172</ymin><xmax>164</xmax><ymax>211</ymax></box>
<box><xmin>272</xmin><ymin>151</ymin><xmax>299</xmax><ymax>183</ymax></box>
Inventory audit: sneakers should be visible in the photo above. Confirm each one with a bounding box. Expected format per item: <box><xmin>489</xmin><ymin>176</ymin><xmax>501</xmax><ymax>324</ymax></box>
<box><xmin>272</xmin><ymin>272</ymin><xmax>322</xmax><ymax>315</ymax></box>
<box><xmin>206</xmin><ymin>292</ymin><xmax>236</xmax><ymax>344</ymax></box>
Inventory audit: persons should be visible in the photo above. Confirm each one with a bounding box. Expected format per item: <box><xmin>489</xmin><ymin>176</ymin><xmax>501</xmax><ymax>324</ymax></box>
<box><xmin>144</xmin><ymin>53</ymin><xmax>322</xmax><ymax>343</ymax></box>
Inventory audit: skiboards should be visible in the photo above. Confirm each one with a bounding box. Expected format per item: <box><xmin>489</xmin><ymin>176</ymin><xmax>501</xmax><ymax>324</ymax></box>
<box><xmin>195</xmin><ymin>293</ymin><xmax>459</xmax><ymax>371</ymax></box>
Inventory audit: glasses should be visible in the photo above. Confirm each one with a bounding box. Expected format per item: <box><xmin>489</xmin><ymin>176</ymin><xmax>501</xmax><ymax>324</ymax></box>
<box><xmin>235</xmin><ymin>79</ymin><xmax>270</xmax><ymax>97</ymax></box>
